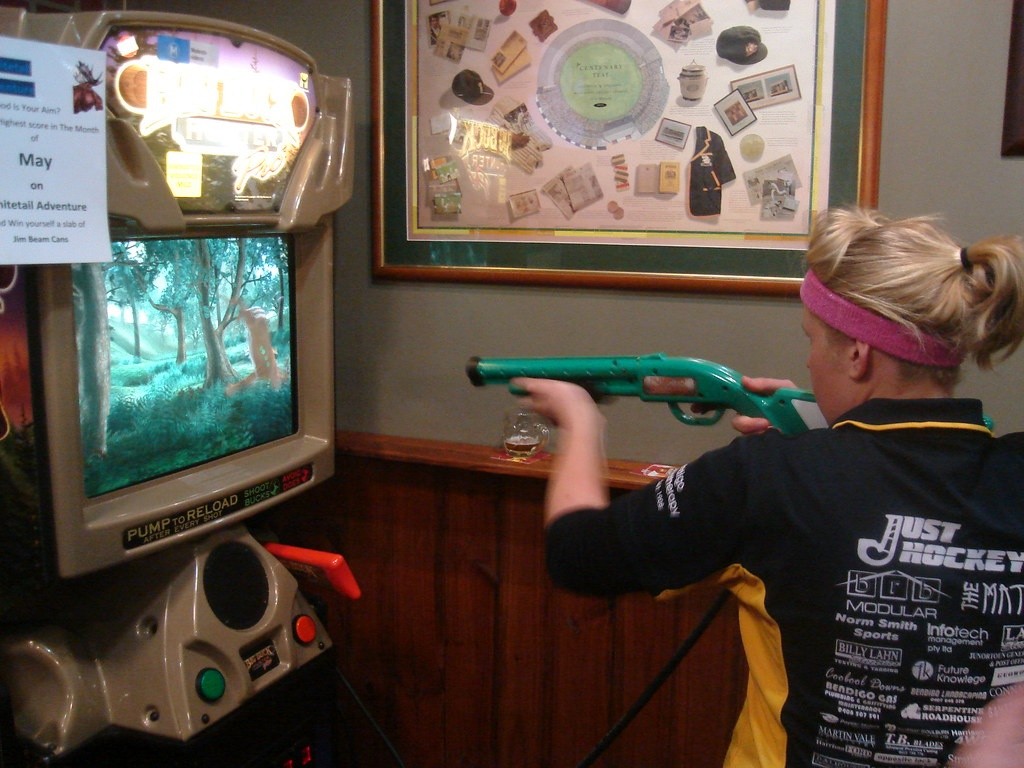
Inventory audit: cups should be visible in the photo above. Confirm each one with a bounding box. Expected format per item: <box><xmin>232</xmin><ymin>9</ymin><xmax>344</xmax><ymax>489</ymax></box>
<box><xmin>503</xmin><ymin>407</ymin><xmax>549</xmax><ymax>457</ymax></box>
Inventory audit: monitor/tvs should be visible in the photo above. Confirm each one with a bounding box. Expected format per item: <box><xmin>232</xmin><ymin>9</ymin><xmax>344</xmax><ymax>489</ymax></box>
<box><xmin>69</xmin><ymin>233</ymin><xmax>299</xmax><ymax>498</ymax></box>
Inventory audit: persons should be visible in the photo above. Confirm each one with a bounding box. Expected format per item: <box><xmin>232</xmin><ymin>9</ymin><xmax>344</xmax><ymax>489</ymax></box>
<box><xmin>513</xmin><ymin>202</ymin><xmax>1024</xmax><ymax>768</ymax></box>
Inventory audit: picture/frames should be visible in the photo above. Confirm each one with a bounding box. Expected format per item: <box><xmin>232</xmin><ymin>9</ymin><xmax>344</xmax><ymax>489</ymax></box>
<box><xmin>369</xmin><ymin>0</ymin><xmax>887</xmax><ymax>298</ymax></box>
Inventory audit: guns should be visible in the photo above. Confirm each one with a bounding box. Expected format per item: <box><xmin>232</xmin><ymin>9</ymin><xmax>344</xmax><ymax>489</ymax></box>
<box><xmin>466</xmin><ymin>353</ymin><xmax>817</xmax><ymax>438</ymax></box>
